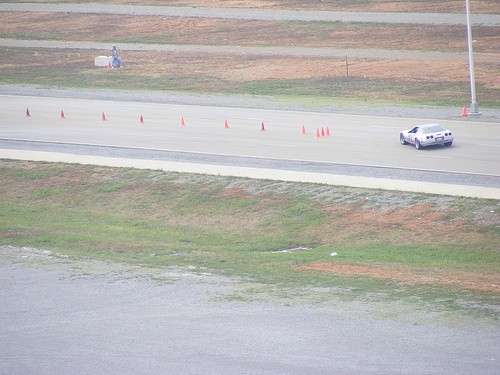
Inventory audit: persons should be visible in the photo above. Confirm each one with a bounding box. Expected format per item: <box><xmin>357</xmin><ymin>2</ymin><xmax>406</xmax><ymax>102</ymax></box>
<box><xmin>111</xmin><ymin>45</ymin><xmax>124</xmax><ymax>68</ymax></box>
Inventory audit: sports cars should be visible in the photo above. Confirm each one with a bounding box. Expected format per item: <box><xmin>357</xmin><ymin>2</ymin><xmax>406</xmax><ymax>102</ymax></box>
<box><xmin>400</xmin><ymin>123</ymin><xmax>454</xmax><ymax>150</ymax></box>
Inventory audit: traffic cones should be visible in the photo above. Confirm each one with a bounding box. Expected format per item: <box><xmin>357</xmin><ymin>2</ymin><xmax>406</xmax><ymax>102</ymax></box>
<box><xmin>59</xmin><ymin>110</ymin><xmax>65</xmax><ymax>119</ymax></box>
<box><xmin>101</xmin><ymin>112</ymin><xmax>106</xmax><ymax>121</ymax></box>
<box><xmin>139</xmin><ymin>114</ymin><xmax>144</xmax><ymax>123</ymax></box>
<box><xmin>260</xmin><ymin>121</ymin><xmax>266</xmax><ymax>131</ymax></box>
<box><xmin>315</xmin><ymin>126</ymin><xmax>331</xmax><ymax>138</ymax></box>
<box><xmin>224</xmin><ymin>119</ymin><xmax>229</xmax><ymax>129</ymax></box>
<box><xmin>25</xmin><ymin>108</ymin><xmax>30</xmax><ymax>116</ymax></box>
<box><xmin>108</xmin><ymin>60</ymin><xmax>112</xmax><ymax>68</ymax></box>
<box><xmin>461</xmin><ymin>106</ymin><xmax>469</xmax><ymax>116</ymax></box>
<box><xmin>180</xmin><ymin>116</ymin><xmax>185</xmax><ymax>125</ymax></box>
<box><xmin>301</xmin><ymin>124</ymin><xmax>307</xmax><ymax>134</ymax></box>
